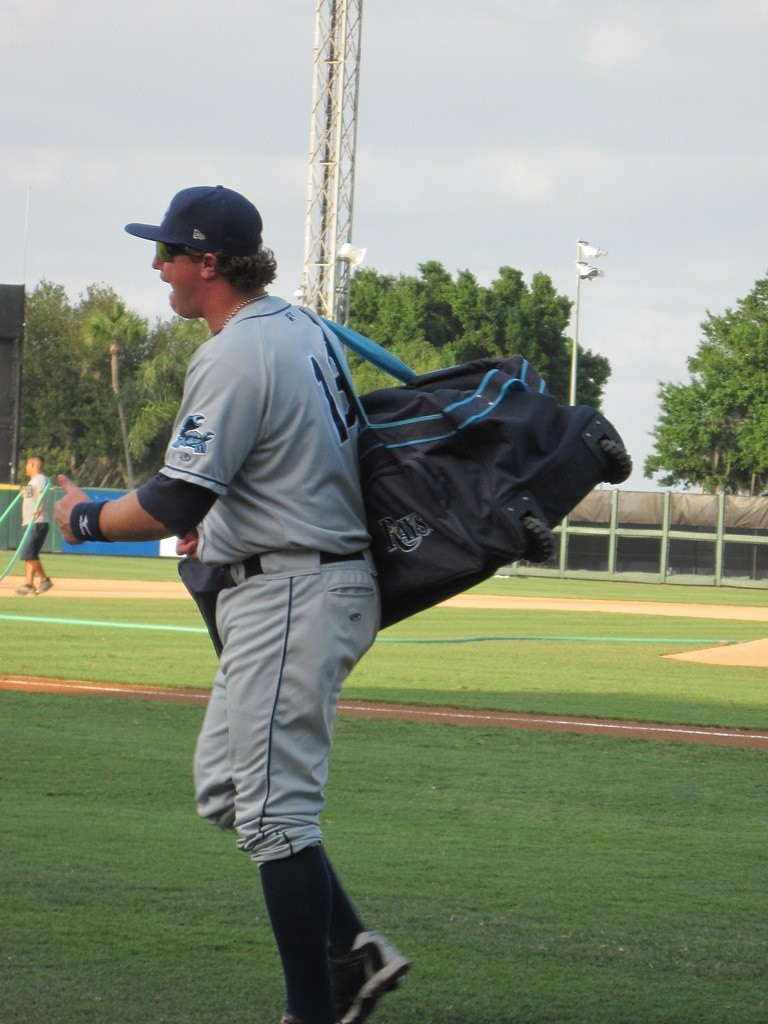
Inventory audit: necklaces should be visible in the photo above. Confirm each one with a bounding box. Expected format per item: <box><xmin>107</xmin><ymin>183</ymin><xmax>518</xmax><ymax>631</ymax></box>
<box><xmin>214</xmin><ymin>293</ymin><xmax>272</xmax><ymax>327</ymax></box>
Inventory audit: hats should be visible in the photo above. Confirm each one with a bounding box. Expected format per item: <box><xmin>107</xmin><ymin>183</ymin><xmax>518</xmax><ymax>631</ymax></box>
<box><xmin>124</xmin><ymin>185</ymin><xmax>263</xmax><ymax>257</ymax></box>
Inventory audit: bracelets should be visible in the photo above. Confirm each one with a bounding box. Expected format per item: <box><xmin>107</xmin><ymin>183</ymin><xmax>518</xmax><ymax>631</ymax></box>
<box><xmin>70</xmin><ymin>500</ymin><xmax>109</xmax><ymax>544</ymax></box>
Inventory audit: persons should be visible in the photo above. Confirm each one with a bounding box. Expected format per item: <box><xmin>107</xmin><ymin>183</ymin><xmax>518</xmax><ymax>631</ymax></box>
<box><xmin>52</xmin><ymin>183</ymin><xmax>411</xmax><ymax>1024</ymax></box>
<box><xmin>16</xmin><ymin>455</ymin><xmax>54</xmax><ymax>596</ymax></box>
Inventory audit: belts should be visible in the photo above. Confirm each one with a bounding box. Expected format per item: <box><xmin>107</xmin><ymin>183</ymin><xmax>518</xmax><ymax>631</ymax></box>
<box><xmin>219</xmin><ymin>548</ymin><xmax>366</xmax><ymax>587</ymax></box>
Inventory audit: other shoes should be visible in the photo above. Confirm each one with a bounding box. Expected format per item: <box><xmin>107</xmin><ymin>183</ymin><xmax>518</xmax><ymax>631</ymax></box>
<box><xmin>34</xmin><ymin>580</ymin><xmax>53</xmax><ymax>594</ymax></box>
<box><xmin>280</xmin><ymin>930</ymin><xmax>411</xmax><ymax>1024</ymax></box>
<box><xmin>15</xmin><ymin>586</ymin><xmax>36</xmax><ymax>595</ymax></box>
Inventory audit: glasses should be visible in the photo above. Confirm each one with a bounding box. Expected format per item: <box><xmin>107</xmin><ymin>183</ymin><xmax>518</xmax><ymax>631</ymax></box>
<box><xmin>155</xmin><ymin>241</ymin><xmax>207</xmax><ymax>262</ymax></box>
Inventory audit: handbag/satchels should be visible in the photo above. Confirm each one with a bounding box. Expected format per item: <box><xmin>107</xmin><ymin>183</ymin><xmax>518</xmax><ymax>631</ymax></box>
<box><xmin>176</xmin><ymin>308</ymin><xmax>633</xmax><ymax>661</ymax></box>
